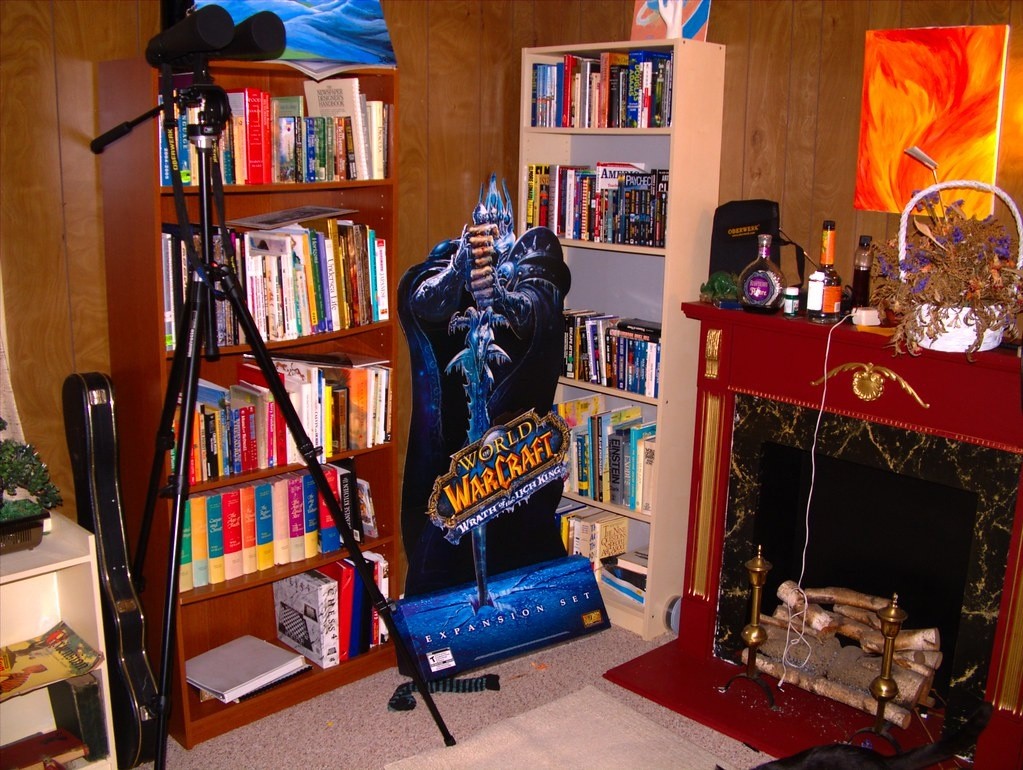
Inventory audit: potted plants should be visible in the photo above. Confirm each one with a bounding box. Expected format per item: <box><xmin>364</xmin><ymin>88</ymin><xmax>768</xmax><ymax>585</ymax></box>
<box><xmin>0</xmin><ymin>437</ymin><xmax>68</xmax><ymax>557</ymax></box>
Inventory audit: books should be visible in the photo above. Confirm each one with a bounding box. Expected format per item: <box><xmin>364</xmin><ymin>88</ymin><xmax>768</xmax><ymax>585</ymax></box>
<box><xmin>159</xmin><ymin>59</ymin><xmax>390</xmax><ymax>187</ymax></box>
<box><xmin>531</xmin><ymin>49</ymin><xmax>673</xmax><ymax>127</ymax></box>
<box><xmin>170</xmin><ymin>353</ymin><xmax>394</xmax><ymax>483</ymax></box>
<box><xmin>186</xmin><ymin>550</ymin><xmax>390</xmax><ymax>704</ymax></box>
<box><xmin>553</xmin><ymin>393</ymin><xmax>656</xmax><ymax>516</ymax></box>
<box><xmin>0</xmin><ymin>672</ymin><xmax>109</xmax><ymax>770</ymax></box>
<box><xmin>161</xmin><ymin>205</ymin><xmax>390</xmax><ymax>351</ymax></box>
<box><xmin>561</xmin><ymin>309</ymin><xmax>662</xmax><ymax>399</ymax></box>
<box><xmin>526</xmin><ymin>162</ymin><xmax>670</xmax><ymax>247</ymax></box>
<box><xmin>178</xmin><ymin>456</ymin><xmax>379</xmax><ymax>592</ymax></box>
<box><xmin>0</xmin><ymin>621</ymin><xmax>104</xmax><ymax>701</ymax></box>
<box><xmin>554</xmin><ymin>503</ymin><xmax>648</xmax><ymax>606</ymax></box>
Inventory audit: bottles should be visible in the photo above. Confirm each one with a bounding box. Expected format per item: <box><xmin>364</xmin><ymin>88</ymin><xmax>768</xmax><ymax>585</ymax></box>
<box><xmin>850</xmin><ymin>236</ymin><xmax>874</xmax><ymax>307</ymax></box>
<box><xmin>737</xmin><ymin>235</ymin><xmax>786</xmax><ymax>316</ymax></box>
<box><xmin>806</xmin><ymin>220</ymin><xmax>842</xmax><ymax>324</ymax></box>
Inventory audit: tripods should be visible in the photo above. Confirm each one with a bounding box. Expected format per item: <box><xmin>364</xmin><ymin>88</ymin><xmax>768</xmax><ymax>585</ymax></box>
<box><xmin>90</xmin><ymin>84</ymin><xmax>455</xmax><ymax>770</ymax></box>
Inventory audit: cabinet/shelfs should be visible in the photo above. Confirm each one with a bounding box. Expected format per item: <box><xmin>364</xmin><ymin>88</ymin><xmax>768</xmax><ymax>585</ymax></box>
<box><xmin>513</xmin><ymin>38</ymin><xmax>726</xmax><ymax>641</ymax></box>
<box><xmin>94</xmin><ymin>48</ymin><xmax>404</xmax><ymax>751</ymax></box>
<box><xmin>0</xmin><ymin>498</ymin><xmax>117</xmax><ymax>770</ymax></box>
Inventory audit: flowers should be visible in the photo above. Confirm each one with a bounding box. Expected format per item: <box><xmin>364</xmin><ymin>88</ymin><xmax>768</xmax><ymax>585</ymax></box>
<box><xmin>861</xmin><ymin>183</ymin><xmax>1023</xmax><ymax>366</ymax></box>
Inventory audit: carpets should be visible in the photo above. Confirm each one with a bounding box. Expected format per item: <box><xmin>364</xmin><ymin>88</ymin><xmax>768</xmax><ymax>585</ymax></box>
<box><xmin>384</xmin><ymin>683</ymin><xmax>743</xmax><ymax>770</ymax></box>
